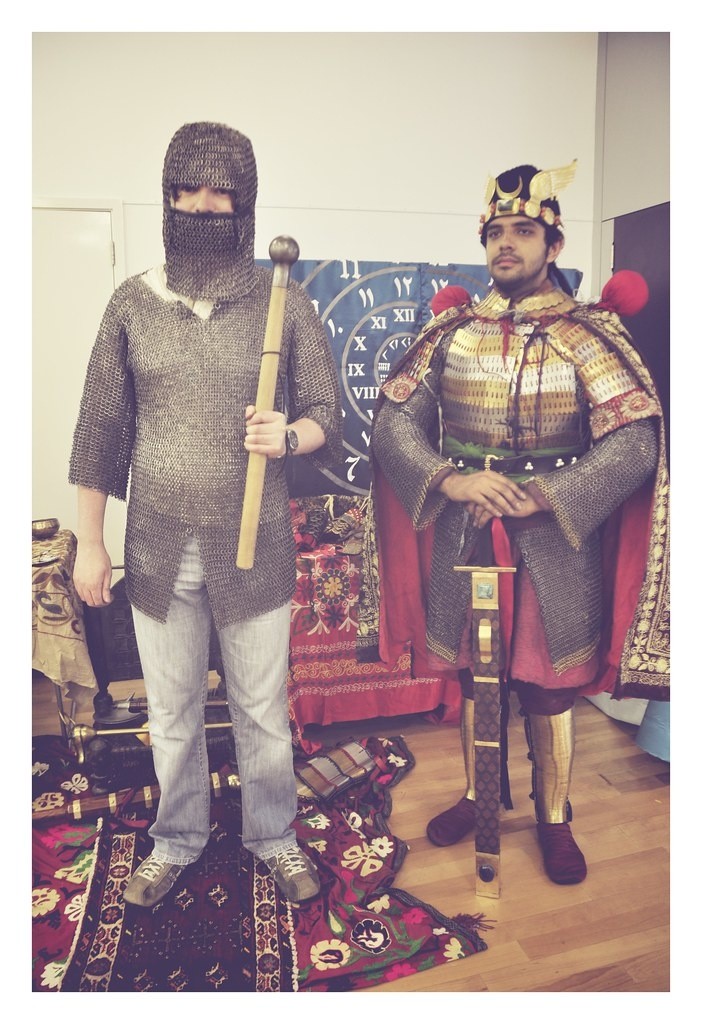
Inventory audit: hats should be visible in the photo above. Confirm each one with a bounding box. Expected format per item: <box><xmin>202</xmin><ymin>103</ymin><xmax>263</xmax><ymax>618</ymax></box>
<box><xmin>479</xmin><ymin>159</ymin><xmax>578</xmax><ymax>247</ymax></box>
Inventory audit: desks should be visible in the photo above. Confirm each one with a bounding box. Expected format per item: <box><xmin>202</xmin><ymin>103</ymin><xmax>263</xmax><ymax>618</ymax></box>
<box><xmin>32</xmin><ymin>529</ymin><xmax>99</xmax><ymax>738</ymax></box>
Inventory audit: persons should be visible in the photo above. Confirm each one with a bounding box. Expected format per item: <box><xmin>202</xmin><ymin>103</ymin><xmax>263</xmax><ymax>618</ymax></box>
<box><xmin>371</xmin><ymin>158</ymin><xmax>670</xmax><ymax>885</ymax></box>
<box><xmin>67</xmin><ymin>121</ymin><xmax>340</xmax><ymax>908</ymax></box>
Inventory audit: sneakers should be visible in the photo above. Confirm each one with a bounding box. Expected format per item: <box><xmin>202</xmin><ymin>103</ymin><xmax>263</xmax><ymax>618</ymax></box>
<box><xmin>122</xmin><ymin>854</ymin><xmax>187</xmax><ymax>907</ymax></box>
<box><xmin>263</xmin><ymin>846</ymin><xmax>321</xmax><ymax>902</ymax></box>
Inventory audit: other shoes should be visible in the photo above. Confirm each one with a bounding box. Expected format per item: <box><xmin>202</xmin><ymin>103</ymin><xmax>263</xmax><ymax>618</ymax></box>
<box><xmin>426</xmin><ymin>795</ymin><xmax>477</xmax><ymax>847</ymax></box>
<box><xmin>536</xmin><ymin>822</ymin><xmax>587</xmax><ymax>885</ymax></box>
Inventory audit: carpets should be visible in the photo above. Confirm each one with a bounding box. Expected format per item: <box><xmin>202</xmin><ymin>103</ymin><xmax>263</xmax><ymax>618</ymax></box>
<box><xmin>32</xmin><ymin>735</ymin><xmax>496</xmax><ymax>992</ymax></box>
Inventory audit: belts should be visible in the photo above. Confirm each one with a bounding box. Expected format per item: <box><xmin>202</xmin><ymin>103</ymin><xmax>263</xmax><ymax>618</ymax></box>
<box><xmin>446</xmin><ymin>453</ymin><xmax>582</xmax><ymax>472</ymax></box>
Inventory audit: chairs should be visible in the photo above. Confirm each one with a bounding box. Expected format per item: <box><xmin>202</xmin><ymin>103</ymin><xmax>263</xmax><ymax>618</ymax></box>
<box><xmin>285</xmin><ymin>495</ymin><xmax>470</xmax><ymax>758</ymax></box>
<box><xmin>83</xmin><ymin>564</ymin><xmax>235</xmax><ymax>796</ymax></box>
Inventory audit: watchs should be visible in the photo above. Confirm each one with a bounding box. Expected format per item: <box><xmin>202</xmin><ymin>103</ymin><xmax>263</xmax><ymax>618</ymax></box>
<box><xmin>276</xmin><ymin>429</ymin><xmax>298</xmax><ymax>460</ymax></box>
